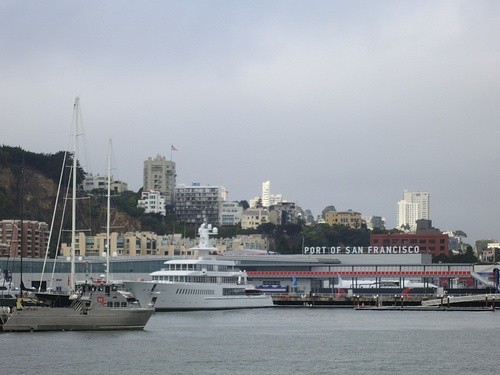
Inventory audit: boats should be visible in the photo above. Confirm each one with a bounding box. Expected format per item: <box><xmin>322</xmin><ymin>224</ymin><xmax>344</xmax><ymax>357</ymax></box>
<box><xmin>0</xmin><ymin>281</ymin><xmax>157</xmax><ymax>328</ymax></box>
<box><xmin>123</xmin><ymin>259</ymin><xmax>276</xmax><ymax>310</ymax></box>
